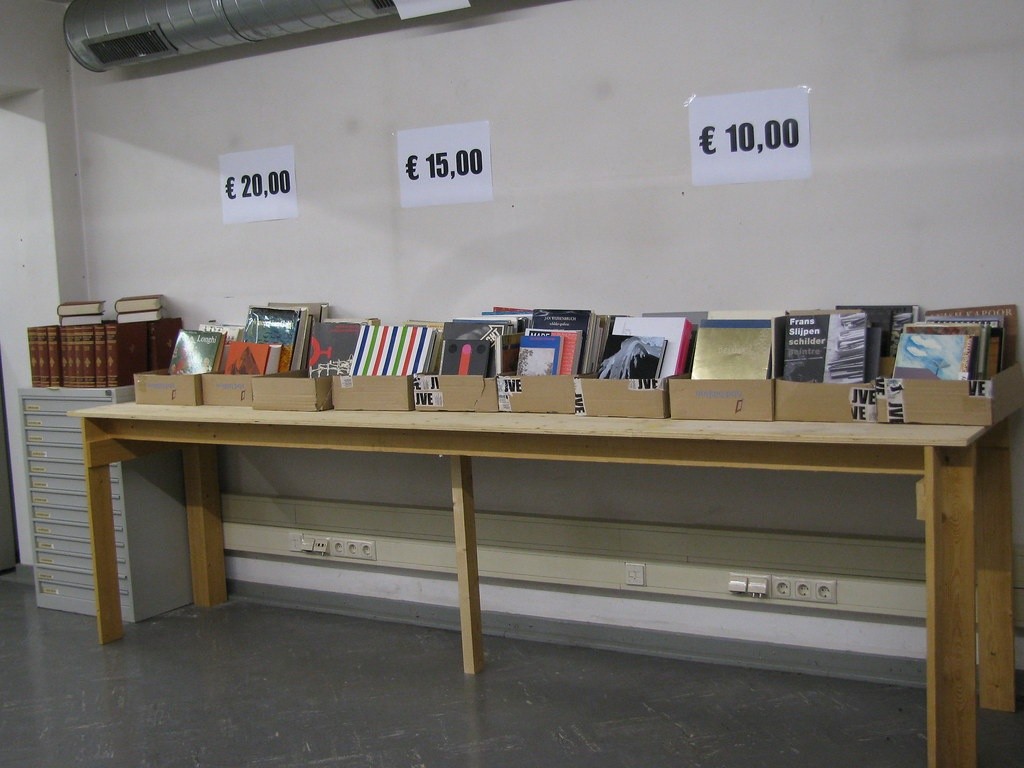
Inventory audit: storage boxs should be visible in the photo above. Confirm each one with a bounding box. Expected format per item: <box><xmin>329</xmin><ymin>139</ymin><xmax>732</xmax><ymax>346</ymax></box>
<box><xmin>330</xmin><ymin>373</ymin><xmax>413</xmax><ymax>413</ymax></box>
<box><xmin>667</xmin><ymin>373</ymin><xmax>776</xmax><ymax>422</ymax></box>
<box><xmin>875</xmin><ymin>363</ymin><xmax>1024</xmax><ymax>427</ymax></box>
<box><xmin>494</xmin><ymin>373</ymin><xmax>573</xmax><ymax>415</ymax></box>
<box><xmin>255</xmin><ymin>371</ymin><xmax>330</xmax><ymax>412</ymax></box>
<box><xmin>134</xmin><ymin>369</ymin><xmax>201</xmax><ymax>407</ymax></box>
<box><xmin>202</xmin><ymin>373</ymin><xmax>259</xmax><ymax>407</ymax></box>
<box><xmin>774</xmin><ymin>378</ymin><xmax>875</xmax><ymax>423</ymax></box>
<box><xmin>574</xmin><ymin>374</ymin><xmax>668</xmax><ymax>419</ymax></box>
<box><xmin>412</xmin><ymin>373</ymin><xmax>498</xmax><ymax>413</ymax></box>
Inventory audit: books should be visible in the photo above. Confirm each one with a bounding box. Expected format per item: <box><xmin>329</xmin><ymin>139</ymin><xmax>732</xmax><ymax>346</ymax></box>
<box><xmin>691</xmin><ymin>305</ymin><xmax>919</xmax><ymax>384</ymax></box>
<box><xmin>438</xmin><ymin>307</ymin><xmax>534</xmax><ymax>376</ymax></box>
<box><xmin>348</xmin><ymin>320</ymin><xmax>448</xmax><ymax>376</ymax></box>
<box><xmin>115</xmin><ymin>295</ymin><xmax>163</xmax><ymax>323</ymax></box>
<box><xmin>516</xmin><ymin>309</ymin><xmax>709</xmax><ymax>379</ymax></box>
<box><xmin>308</xmin><ymin>318</ymin><xmax>382</xmax><ymax>378</ymax></box>
<box><xmin>27</xmin><ymin>318</ymin><xmax>182</xmax><ymax>388</ymax></box>
<box><xmin>57</xmin><ymin>301</ymin><xmax>105</xmax><ymax>327</ymax></box>
<box><xmin>167</xmin><ymin>329</ymin><xmax>228</xmax><ymax>375</ymax></box>
<box><xmin>892</xmin><ymin>304</ymin><xmax>1017</xmax><ymax>382</ymax></box>
<box><xmin>198</xmin><ymin>324</ymin><xmax>244</xmax><ymax>374</ymax></box>
<box><xmin>224</xmin><ymin>303</ymin><xmax>330</xmax><ymax>375</ymax></box>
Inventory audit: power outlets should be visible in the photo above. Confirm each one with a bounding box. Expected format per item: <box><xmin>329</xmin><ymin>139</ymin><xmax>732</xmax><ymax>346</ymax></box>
<box><xmin>727</xmin><ymin>572</ymin><xmax>838</xmax><ymax>605</ymax></box>
<box><xmin>287</xmin><ymin>532</ymin><xmax>377</xmax><ymax>560</ymax></box>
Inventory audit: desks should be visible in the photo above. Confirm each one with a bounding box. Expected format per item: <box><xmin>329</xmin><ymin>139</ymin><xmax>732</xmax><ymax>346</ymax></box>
<box><xmin>64</xmin><ymin>402</ymin><xmax>1014</xmax><ymax>768</ymax></box>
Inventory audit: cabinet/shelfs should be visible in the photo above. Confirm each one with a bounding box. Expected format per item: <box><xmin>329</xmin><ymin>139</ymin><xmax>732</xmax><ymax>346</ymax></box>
<box><xmin>16</xmin><ymin>385</ymin><xmax>194</xmax><ymax>624</ymax></box>
